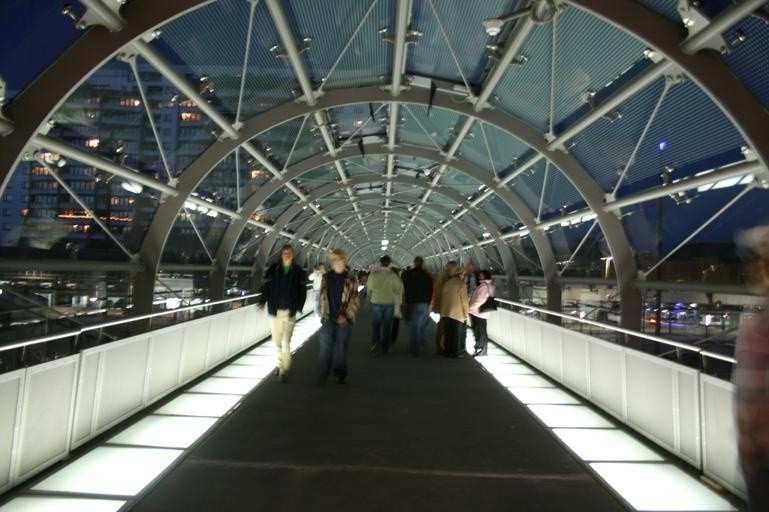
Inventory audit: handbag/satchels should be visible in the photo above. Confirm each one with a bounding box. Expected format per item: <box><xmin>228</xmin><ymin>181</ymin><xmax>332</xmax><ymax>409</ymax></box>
<box><xmin>479</xmin><ymin>297</ymin><xmax>497</xmax><ymax>312</ymax></box>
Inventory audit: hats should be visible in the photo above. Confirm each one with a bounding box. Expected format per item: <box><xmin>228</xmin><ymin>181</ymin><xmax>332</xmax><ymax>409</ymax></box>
<box><xmin>449</xmin><ymin>267</ymin><xmax>466</xmax><ymax>277</ymax></box>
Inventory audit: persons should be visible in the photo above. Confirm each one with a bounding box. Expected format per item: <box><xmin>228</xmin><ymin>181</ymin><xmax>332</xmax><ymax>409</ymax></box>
<box><xmin>730</xmin><ymin>224</ymin><xmax>769</xmax><ymax>512</ymax></box>
<box><xmin>257</xmin><ymin>244</ymin><xmax>497</xmax><ymax>387</ymax></box>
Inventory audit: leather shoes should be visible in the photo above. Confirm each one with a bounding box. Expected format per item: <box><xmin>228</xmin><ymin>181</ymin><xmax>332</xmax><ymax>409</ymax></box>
<box><xmin>439</xmin><ymin>349</ymin><xmax>487</xmax><ymax>359</ymax></box>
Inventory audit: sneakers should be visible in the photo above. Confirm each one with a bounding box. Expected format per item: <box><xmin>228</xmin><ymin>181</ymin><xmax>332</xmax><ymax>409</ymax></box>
<box><xmin>320</xmin><ymin>376</ymin><xmax>345</xmax><ymax>385</ymax></box>
<box><xmin>274</xmin><ymin>366</ymin><xmax>287</xmax><ymax>384</ymax></box>
<box><xmin>369</xmin><ymin>342</ymin><xmax>389</xmax><ymax>353</ymax></box>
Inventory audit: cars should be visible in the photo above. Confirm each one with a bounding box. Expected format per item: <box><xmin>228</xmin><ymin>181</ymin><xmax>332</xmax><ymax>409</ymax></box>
<box><xmin>644</xmin><ymin>303</ymin><xmax>738</xmax><ymax>328</ymax></box>
<box><xmin>92</xmin><ymin>296</ymin><xmax>211</xmax><ymax>309</ymax></box>
